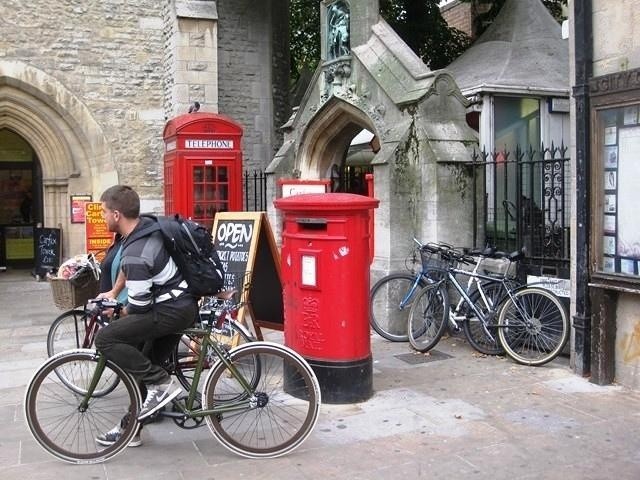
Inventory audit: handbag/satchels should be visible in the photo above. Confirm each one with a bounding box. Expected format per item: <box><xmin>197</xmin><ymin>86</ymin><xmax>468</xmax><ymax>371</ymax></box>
<box><xmin>57</xmin><ymin>253</ymin><xmax>101</xmax><ymax>280</ymax></box>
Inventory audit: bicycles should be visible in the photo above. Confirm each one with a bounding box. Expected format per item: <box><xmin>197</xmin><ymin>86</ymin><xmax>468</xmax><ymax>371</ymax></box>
<box><xmin>407</xmin><ymin>249</ymin><xmax>570</xmax><ymax>367</ymax></box>
<box><xmin>24</xmin><ymin>298</ymin><xmax>323</xmax><ymax>467</ymax></box>
<box><xmin>369</xmin><ymin>237</ymin><xmax>529</xmax><ymax>356</ymax></box>
<box><xmin>46</xmin><ymin>245</ymin><xmax>261</xmax><ymax>406</ymax></box>
<box><xmin>518</xmin><ymin>208</ymin><xmax>570</xmax><ymax>257</ymax></box>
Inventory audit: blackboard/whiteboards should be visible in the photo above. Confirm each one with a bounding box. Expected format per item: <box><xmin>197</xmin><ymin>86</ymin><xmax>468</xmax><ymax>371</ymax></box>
<box><xmin>197</xmin><ymin>212</ymin><xmax>263</xmax><ymax>345</ymax></box>
<box><xmin>33</xmin><ymin>228</ymin><xmax>63</xmax><ymax>269</ymax></box>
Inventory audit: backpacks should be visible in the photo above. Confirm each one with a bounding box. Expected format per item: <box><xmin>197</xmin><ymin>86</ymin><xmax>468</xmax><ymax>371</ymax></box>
<box><xmin>120</xmin><ymin>214</ymin><xmax>226</xmax><ymax>297</ymax></box>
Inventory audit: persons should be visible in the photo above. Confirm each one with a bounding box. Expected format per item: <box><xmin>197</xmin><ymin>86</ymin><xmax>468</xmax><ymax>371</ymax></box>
<box><xmin>20</xmin><ymin>191</ymin><xmax>33</xmax><ymax>225</ymax></box>
<box><xmin>326</xmin><ymin>4</ymin><xmax>350</xmax><ymax>58</ymax></box>
<box><xmin>95</xmin><ymin>186</ymin><xmax>198</xmax><ymax>447</ymax></box>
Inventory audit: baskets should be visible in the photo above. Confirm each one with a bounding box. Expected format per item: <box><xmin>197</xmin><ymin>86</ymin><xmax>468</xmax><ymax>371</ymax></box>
<box><xmin>421</xmin><ymin>251</ymin><xmax>458</xmax><ymax>282</ymax></box>
<box><xmin>48</xmin><ymin>275</ymin><xmax>97</xmax><ymax>310</ymax></box>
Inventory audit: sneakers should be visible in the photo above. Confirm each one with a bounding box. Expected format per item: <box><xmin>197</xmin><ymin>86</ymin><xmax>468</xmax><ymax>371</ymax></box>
<box><xmin>95</xmin><ymin>425</ymin><xmax>142</xmax><ymax>446</ymax></box>
<box><xmin>137</xmin><ymin>375</ymin><xmax>183</xmax><ymax>420</ymax></box>
